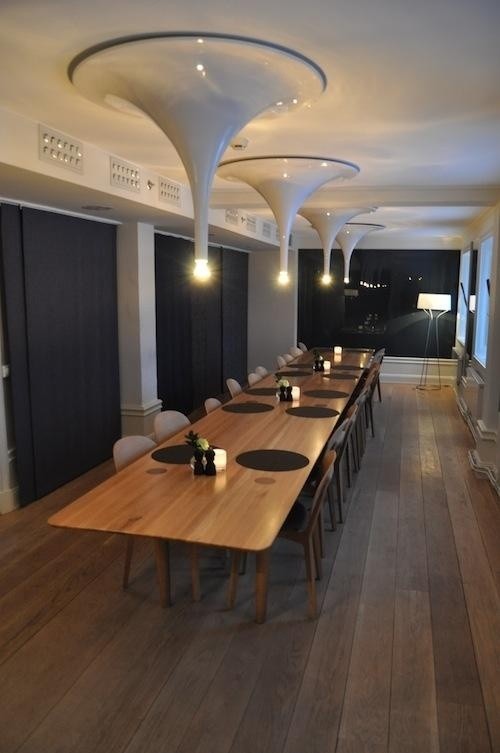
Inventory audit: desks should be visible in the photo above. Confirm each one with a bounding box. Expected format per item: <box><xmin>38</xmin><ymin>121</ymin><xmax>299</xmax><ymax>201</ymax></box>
<box><xmin>49</xmin><ymin>347</ymin><xmax>376</xmax><ymax>614</ymax></box>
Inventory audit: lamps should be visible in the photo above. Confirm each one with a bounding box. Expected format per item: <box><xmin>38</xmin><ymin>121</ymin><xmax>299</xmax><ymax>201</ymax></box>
<box><xmin>333</xmin><ymin>219</ymin><xmax>386</xmax><ymax>285</ymax></box>
<box><xmin>418</xmin><ymin>290</ymin><xmax>453</xmax><ymax>395</ymax></box>
<box><xmin>213</xmin><ymin>152</ymin><xmax>362</xmax><ymax>288</ymax></box>
<box><xmin>66</xmin><ymin>29</ymin><xmax>329</xmax><ymax>285</ymax></box>
<box><xmin>297</xmin><ymin>203</ymin><xmax>378</xmax><ymax>287</ymax></box>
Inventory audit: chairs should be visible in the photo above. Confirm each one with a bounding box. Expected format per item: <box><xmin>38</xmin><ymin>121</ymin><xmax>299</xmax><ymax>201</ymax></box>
<box><xmin>153</xmin><ymin>410</ymin><xmax>192</xmax><ymax>442</ymax></box>
<box><xmin>203</xmin><ymin>340</ymin><xmax>385</xmax><ymax>579</ymax></box>
<box><xmin>113</xmin><ymin>435</ymin><xmax>223</xmax><ymax>605</ymax></box>
<box><xmin>228</xmin><ymin>450</ymin><xmax>339</xmax><ymax>621</ymax></box>
